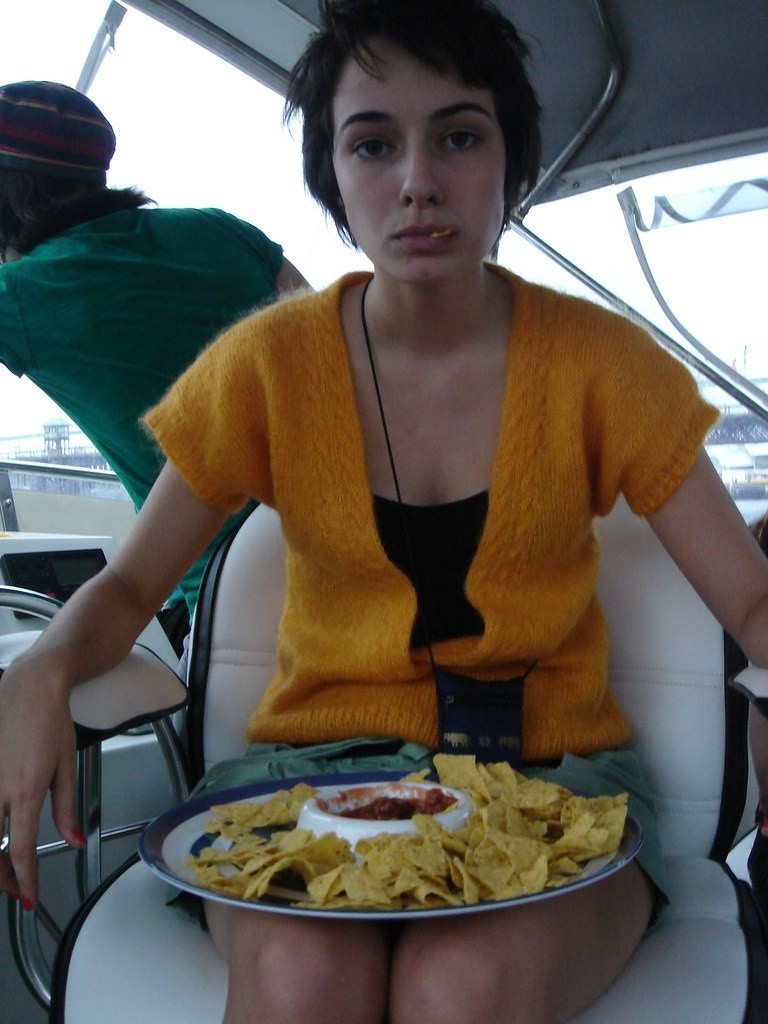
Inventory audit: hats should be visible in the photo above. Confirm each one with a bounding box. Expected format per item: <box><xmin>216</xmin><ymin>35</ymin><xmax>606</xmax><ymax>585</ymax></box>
<box><xmin>0</xmin><ymin>80</ymin><xmax>116</xmax><ymax>183</ymax></box>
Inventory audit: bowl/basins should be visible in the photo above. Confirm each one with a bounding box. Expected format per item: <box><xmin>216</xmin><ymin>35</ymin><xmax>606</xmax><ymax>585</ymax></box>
<box><xmin>296</xmin><ymin>780</ymin><xmax>474</xmax><ymax>864</ymax></box>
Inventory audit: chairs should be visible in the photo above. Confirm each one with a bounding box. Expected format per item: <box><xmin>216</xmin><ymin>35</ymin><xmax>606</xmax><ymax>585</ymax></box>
<box><xmin>0</xmin><ymin>493</ymin><xmax>768</xmax><ymax>1024</ymax></box>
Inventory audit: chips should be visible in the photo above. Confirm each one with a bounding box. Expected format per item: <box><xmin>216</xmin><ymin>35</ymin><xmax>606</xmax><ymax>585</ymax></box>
<box><xmin>186</xmin><ymin>752</ymin><xmax>632</xmax><ymax>908</ymax></box>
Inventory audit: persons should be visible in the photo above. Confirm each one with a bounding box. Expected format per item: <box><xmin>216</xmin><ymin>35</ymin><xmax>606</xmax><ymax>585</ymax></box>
<box><xmin>1</xmin><ymin>79</ymin><xmax>321</xmax><ymax>746</ymax></box>
<box><xmin>0</xmin><ymin>0</ymin><xmax>768</xmax><ymax>1024</ymax></box>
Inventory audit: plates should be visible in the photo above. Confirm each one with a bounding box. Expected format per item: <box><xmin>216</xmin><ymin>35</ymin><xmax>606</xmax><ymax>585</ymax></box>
<box><xmin>136</xmin><ymin>769</ymin><xmax>643</xmax><ymax>920</ymax></box>
